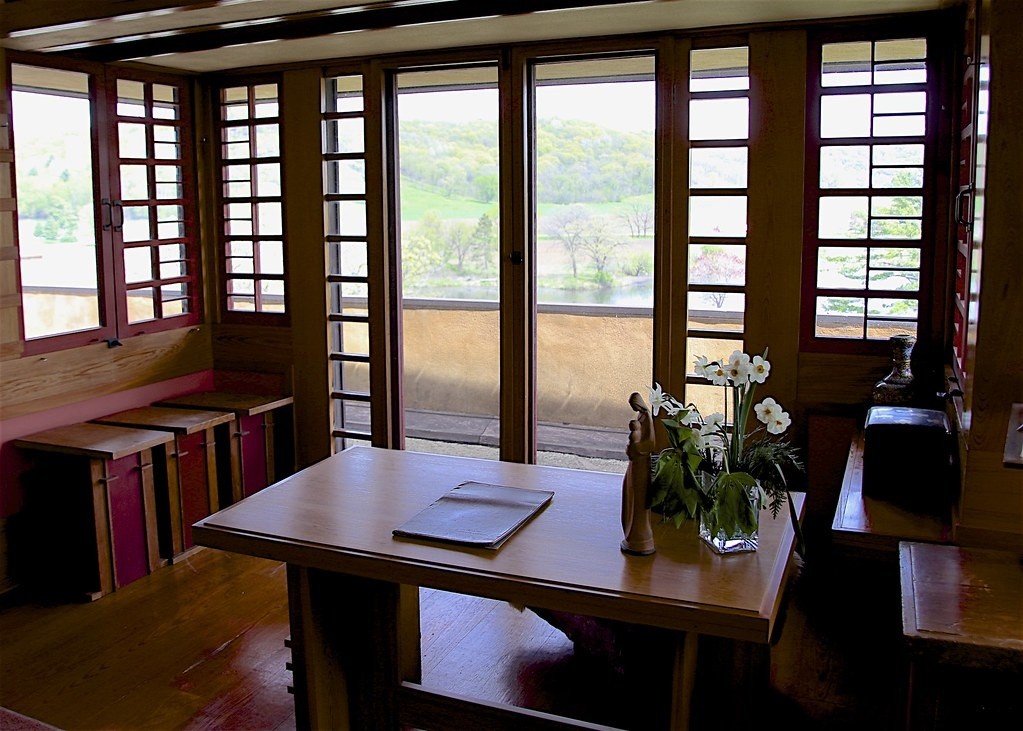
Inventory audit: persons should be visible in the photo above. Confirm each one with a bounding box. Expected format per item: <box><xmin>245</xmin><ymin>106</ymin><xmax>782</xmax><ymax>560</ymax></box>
<box><xmin>620</xmin><ymin>392</ymin><xmax>656</xmax><ymax>553</ymax></box>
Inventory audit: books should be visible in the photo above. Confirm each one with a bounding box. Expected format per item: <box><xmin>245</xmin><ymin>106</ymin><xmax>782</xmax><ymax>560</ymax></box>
<box><xmin>392</xmin><ymin>480</ymin><xmax>555</xmax><ymax>550</ymax></box>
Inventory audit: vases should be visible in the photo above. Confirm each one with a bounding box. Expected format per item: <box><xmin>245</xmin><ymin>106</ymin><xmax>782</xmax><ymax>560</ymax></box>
<box><xmin>699</xmin><ymin>467</ymin><xmax>760</xmax><ymax>552</ymax></box>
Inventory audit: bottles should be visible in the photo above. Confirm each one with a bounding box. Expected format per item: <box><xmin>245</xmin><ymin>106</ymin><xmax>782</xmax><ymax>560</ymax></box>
<box><xmin>873</xmin><ymin>334</ymin><xmax>927</xmax><ymax>408</ymax></box>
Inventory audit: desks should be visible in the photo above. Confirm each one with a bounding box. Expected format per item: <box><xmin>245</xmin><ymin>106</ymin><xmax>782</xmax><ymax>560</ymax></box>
<box><xmin>833</xmin><ymin>429</ymin><xmax>953</xmax><ymax>541</ymax></box>
<box><xmin>191</xmin><ymin>447</ymin><xmax>807</xmax><ymax>731</ymax></box>
<box><xmin>895</xmin><ymin>541</ymin><xmax>1023</xmax><ymax>731</ymax></box>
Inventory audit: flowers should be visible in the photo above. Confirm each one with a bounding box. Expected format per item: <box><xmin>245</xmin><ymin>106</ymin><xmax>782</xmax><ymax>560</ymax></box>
<box><xmin>641</xmin><ymin>350</ymin><xmax>805</xmax><ymax>535</ymax></box>
